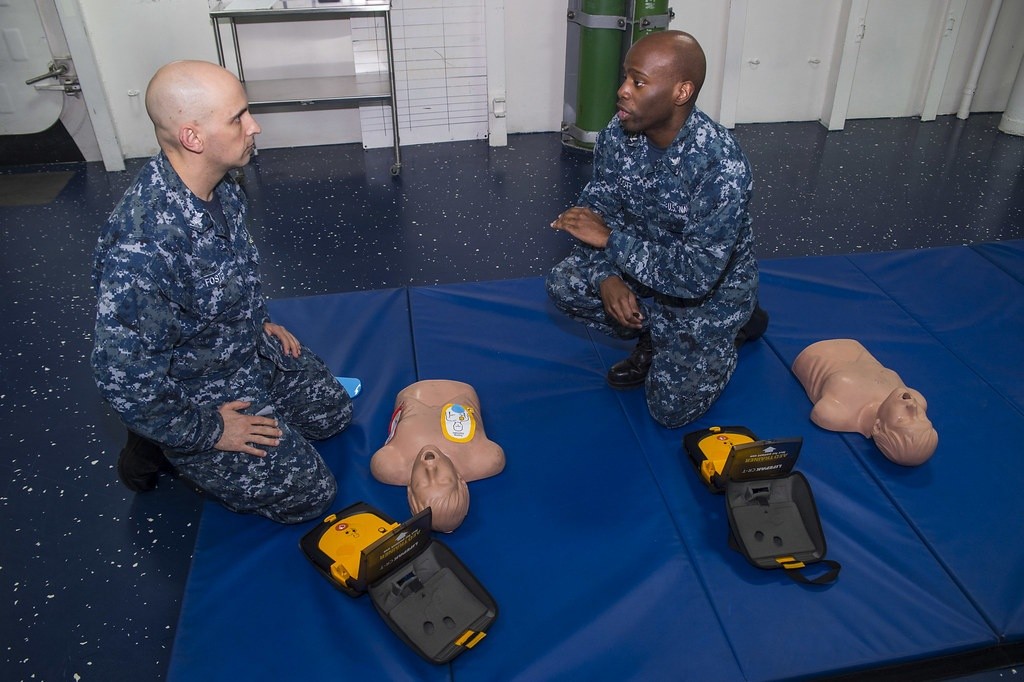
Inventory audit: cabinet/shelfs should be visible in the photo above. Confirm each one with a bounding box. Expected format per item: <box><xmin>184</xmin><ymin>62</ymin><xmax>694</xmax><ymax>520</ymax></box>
<box><xmin>209</xmin><ymin>0</ymin><xmax>402</xmax><ymax>185</ymax></box>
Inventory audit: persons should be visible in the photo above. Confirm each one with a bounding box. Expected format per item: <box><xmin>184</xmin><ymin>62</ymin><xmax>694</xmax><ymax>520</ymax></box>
<box><xmin>792</xmin><ymin>338</ymin><xmax>938</xmax><ymax>465</ymax></box>
<box><xmin>370</xmin><ymin>379</ymin><xmax>506</xmax><ymax>533</ymax></box>
<box><xmin>544</xmin><ymin>30</ymin><xmax>768</xmax><ymax>426</ymax></box>
<box><xmin>92</xmin><ymin>60</ymin><xmax>353</xmax><ymax>523</ymax></box>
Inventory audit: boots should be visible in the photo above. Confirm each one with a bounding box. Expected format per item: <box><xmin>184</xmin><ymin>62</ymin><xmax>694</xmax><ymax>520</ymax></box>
<box><xmin>734</xmin><ymin>300</ymin><xmax>769</xmax><ymax>348</ymax></box>
<box><xmin>608</xmin><ymin>327</ymin><xmax>652</xmax><ymax>388</ymax></box>
<box><xmin>118</xmin><ymin>429</ymin><xmax>174</xmax><ymax>494</ymax></box>
